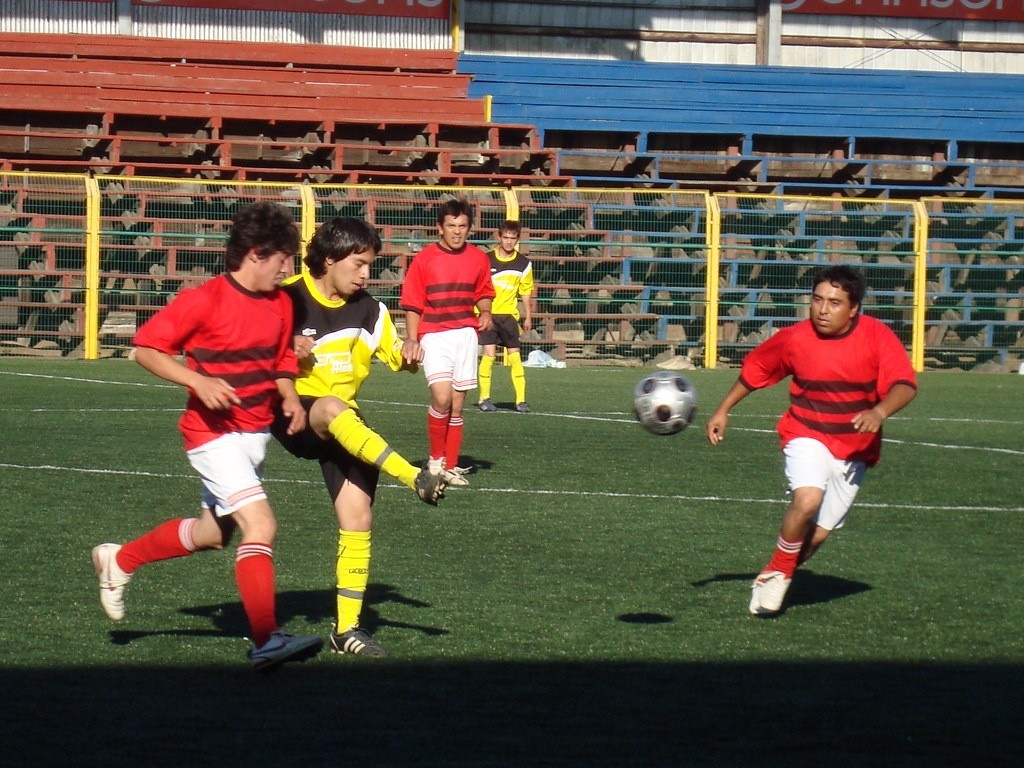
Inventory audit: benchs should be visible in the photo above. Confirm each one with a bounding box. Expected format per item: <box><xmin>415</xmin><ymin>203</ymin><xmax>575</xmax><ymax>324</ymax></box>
<box><xmin>0</xmin><ymin>32</ymin><xmax>1024</xmax><ymax>372</ymax></box>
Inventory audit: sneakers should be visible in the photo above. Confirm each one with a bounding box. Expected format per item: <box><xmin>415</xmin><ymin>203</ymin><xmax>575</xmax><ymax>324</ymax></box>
<box><xmin>243</xmin><ymin>630</ymin><xmax>323</xmax><ymax>672</ymax></box>
<box><xmin>429</xmin><ymin>456</ymin><xmax>444</xmax><ymax>477</ymax></box>
<box><xmin>440</xmin><ymin>470</ymin><xmax>469</xmax><ymax>487</ymax></box>
<box><xmin>328</xmin><ymin>622</ymin><xmax>387</xmax><ymax>660</ymax></box>
<box><xmin>91</xmin><ymin>543</ymin><xmax>135</xmax><ymax>621</ymax></box>
<box><xmin>749</xmin><ymin>570</ymin><xmax>794</xmax><ymax>616</ymax></box>
<box><xmin>414</xmin><ymin>469</ymin><xmax>447</xmax><ymax>506</ymax></box>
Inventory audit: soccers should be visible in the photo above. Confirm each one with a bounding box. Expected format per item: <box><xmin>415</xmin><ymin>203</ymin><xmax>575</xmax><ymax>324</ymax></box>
<box><xmin>634</xmin><ymin>369</ymin><xmax>697</xmax><ymax>436</ymax></box>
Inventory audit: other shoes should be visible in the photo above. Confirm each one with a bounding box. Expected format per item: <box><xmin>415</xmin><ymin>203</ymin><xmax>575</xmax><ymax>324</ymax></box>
<box><xmin>478</xmin><ymin>398</ymin><xmax>497</xmax><ymax>412</ymax></box>
<box><xmin>516</xmin><ymin>401</ymin><xmax>531</xmax><ymax>412</ymax></box>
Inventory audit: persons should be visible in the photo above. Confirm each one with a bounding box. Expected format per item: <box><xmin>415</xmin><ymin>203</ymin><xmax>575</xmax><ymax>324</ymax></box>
<box><xmin>271</xmin><ymin>216</ymin><xmax>447</xmax><ymax>657</ymax></box>
<box><xmin>473</xmin><ymin>220</ymin><xmax>534</xmax><ymax>412</ymax></box>
<box><xmin>399</xmin><ymin>200</ymin><xmax>495</xmax><ymax>485</ymax></box>
<box><xmin>93</xmin><ymin>203</ymin><xmax>306</xmax><ymax>668</ymax></box>
<box><xmin>707</xmin><ymin>266</ymin><xmax>917</xmax><ymax>613</ymax></box>
